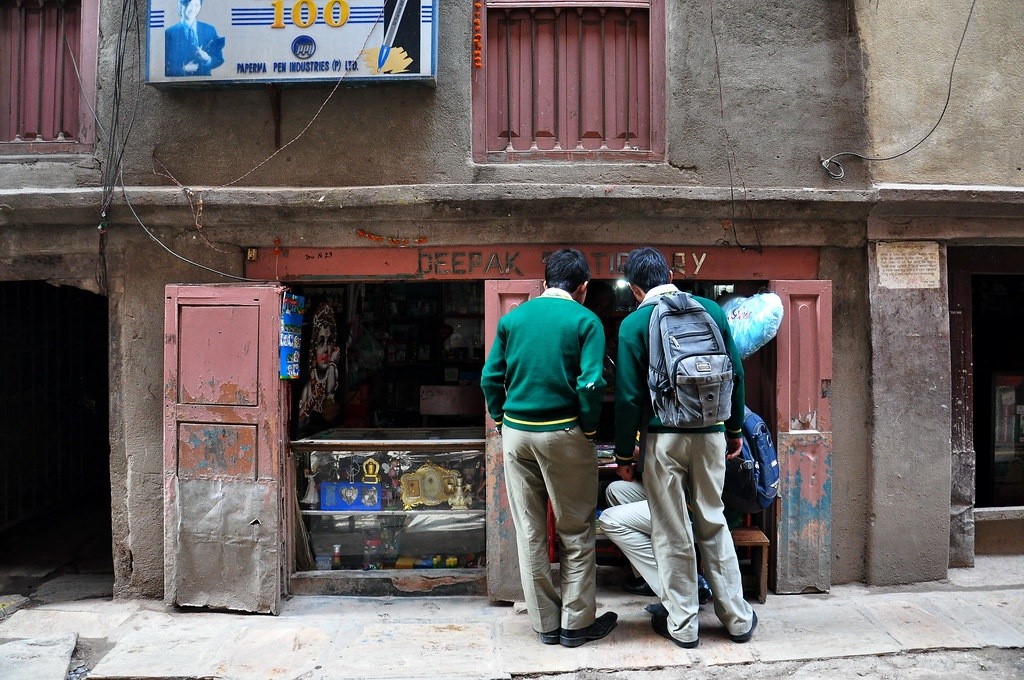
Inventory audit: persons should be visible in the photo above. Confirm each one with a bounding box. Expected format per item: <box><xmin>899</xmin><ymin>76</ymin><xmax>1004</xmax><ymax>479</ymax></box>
<box><xmin>599</xmin><ymin>250</ymin><xmax>758</xmax><ymax>648</ymax></box>
<box><xmin>302</xmin><ymin>467</ymin><xmax>320</xmax><ymax>503</ymax></box>
<box><xmin>481</xmin><ymin>249</ymin><xmax>617</xmax><ymax>646</ymax></box>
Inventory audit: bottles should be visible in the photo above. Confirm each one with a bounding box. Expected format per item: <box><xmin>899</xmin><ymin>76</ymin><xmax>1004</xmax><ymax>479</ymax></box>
<box><xmin>332</xmin><ymin>537</ymin><xmax>384</xmax><ymax>571</ymax></box>
<box><xmin>433</xmin><ymin>555</ymin><xmax>457</xmax><ymax>568</ymax></box>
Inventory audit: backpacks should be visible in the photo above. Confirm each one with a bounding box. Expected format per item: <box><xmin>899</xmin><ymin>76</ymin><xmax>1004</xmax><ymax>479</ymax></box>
<box><xmin>637</xmin><ymin>293</ymin><xmax>735</xmax><ymax>429</ymax></box>
<box><xmin>728</xmin><ymin>406</ymin><xmax>778</xmax><ymax>513</ymax></box>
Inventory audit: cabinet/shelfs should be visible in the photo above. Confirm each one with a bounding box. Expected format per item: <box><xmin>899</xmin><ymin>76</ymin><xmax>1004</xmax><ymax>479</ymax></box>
<box><xmin>291</xmin><ymin>427</ymin><xmax>486</xmax><ymax>596</ymax></box>
<box><xmin>548</xmin><ymin>446</ymin><xmax>638</xmax><ymax>585</ymax></box>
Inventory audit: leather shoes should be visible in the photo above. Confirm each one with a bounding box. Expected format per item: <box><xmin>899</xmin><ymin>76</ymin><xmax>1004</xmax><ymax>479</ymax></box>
<box><xmin>560</xmin><ymin>612</ymin><xmax>618</xmax><ymax>647</ymax></box>
<box><xmin>652</xmin><ymin>613</ymin><xmax>698</xmax><ymax>647</ymax></box>
<box><xmin>623</xmin><ymin>577</ymin><xmax>656</xmax><ymax>596</ymax></box>
<box><xmin>534</xmin><ymin>627</ymin><xmax>559</xmax><ymax>644</ymax></box>
<box><xmin>732</xmin><ymin>609</ymin><xmax>758</xmax><ymax>642</ymax></box>
<box><xmin>647</xmin><ymin>578</ymin><xmax>711</xmax><ymax>613</ymax></box>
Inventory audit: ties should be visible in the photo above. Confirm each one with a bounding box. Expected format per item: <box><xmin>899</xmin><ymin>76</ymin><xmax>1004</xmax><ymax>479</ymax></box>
<box><xmin>188</xmin><ymin>27</ymin><xmax>195</xmax><ymax>44</ymax></box>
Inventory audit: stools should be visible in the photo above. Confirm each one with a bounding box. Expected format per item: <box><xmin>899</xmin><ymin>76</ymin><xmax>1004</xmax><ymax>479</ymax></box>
<box><xmin>702</xmin><ymin>529</ymin><xmax>770</xmax><ymax>602</ymax></box>
